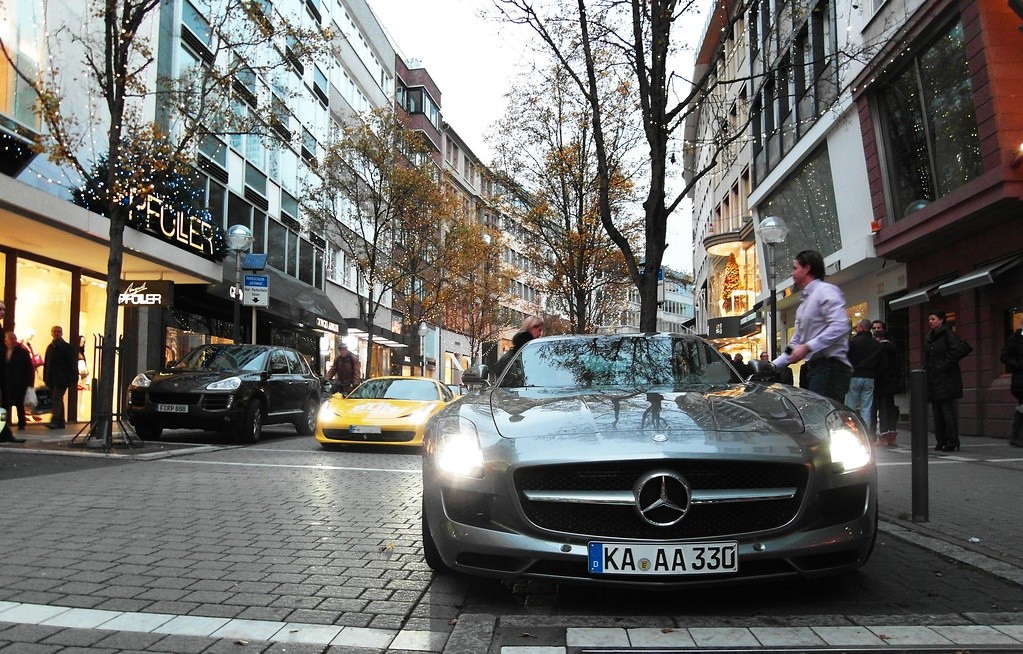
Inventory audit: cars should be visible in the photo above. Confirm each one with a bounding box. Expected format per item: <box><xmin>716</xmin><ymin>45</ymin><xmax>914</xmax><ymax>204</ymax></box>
<box><xmin>448</xmin><ymin>384</ymin><xmax>469</xmax><ymax>398</ymax></box>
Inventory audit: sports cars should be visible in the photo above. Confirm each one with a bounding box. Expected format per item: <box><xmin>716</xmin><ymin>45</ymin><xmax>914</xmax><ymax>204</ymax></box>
<box><xmin>421</xmin><ymin>331</ymin><xmax>879</xmax><ymax>586</ymax></box>
<box><xmin>315</xmin><ymin>376</ymin><xmax>464</xmax><ymax>449</ymax></box>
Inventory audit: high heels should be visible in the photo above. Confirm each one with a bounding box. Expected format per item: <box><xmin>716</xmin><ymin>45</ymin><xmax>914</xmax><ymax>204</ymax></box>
<box><xmin>934</xmin><ymin>439</ymin><xmax>960</xmax><ymax>452</ymax></box>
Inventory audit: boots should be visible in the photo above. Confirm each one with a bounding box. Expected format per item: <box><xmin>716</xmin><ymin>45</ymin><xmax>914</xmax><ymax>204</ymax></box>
<box><xmin>1009</xmin><ymin>410</ymin><xmax>1023</xmax><ymax>448</ymax></box>
<box><xmin>874</xmin><ymin>431</ymin><xmax>898</xmax><ymax>448</ymax></box>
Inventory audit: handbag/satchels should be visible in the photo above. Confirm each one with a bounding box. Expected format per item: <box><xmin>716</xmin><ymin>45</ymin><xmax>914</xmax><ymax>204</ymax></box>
<box><xmin>945</xmin><ymin>329</ymin><xmax>973</xmax><ymax>361</ymax></box>
<box><xmin>32</xmin><ymin>387</ymin><xmax>54</xmax><ymax>414</ymax></box>
<box><xmin>31</xmin><ymin>353</ymin><xmax>43</xmax><ymax>370</ymax></box>
<box><xmin>878</xmin><ymin>406</ymin><xmax>900</xmax><ymax>433</ymax></box>
<box><xmin>24</xmin><ymin>386</ymin><xmax>38</xmax><ymax>407</ymax></box>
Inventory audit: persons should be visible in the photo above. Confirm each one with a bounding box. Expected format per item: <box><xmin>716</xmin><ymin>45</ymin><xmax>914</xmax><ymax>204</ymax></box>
<box><xmin>43</xmin><ymin>325</ymin><xmax>73</xmax><ymax>429</ymax></box>
<box><xmin>769</xmin><ymin>250</ymin><xmax>853</xmax><ymax>403</ymax></box>
<box><xmin>1000</xmin><ymin>327</ymin><xmax>1023</xmax><ymax>447</ymax></box>
<box><xmin>492</xmin><ymin>314</ymin><xmax>543</xmax><ymax>378</ymax></box>
<box><xmin>844</xmin><ymin>319</ymin><xmax>900</xmax><ymax>448</ymax></box>
<box><xmin>0</xmin><ymin>300</ymin><xmax>34</xmax><ymax>443</ymax></box>
<box><xmin>78</xmin><ymin>336</ymin><xmax>89</xmax><ymax>421</ymax></box>
<box><xmin>922</xmin><ymin>311</ymin><xmax>963</xmax><ymax>450</ymax></box>
<box><xmin>722</xmin><ymin>352</ymin><xmax>749</xmax><ymax>383</ymax></box>
<box><xmin>18</xmin><ymin>327</ymin><xmax>44</xmax><ymax>422</ymax></box>
<box><xmin>760</xmin><ymin>352</ymin><xmax>771</xmax><ymax>376</ymax></box>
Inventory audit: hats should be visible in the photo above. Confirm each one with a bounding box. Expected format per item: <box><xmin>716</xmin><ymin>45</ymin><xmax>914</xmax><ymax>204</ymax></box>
<box><xmin>337</xmin><ymin>343</ymin><xmax>349</xmax><ymax>348</ymax></box>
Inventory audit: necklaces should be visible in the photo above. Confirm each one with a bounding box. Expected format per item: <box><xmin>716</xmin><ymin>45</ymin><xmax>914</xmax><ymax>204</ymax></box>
<box><xmin>321</xmin><ymin>343</ymin><xmax>361</xmax><ymax>394</ymax></box>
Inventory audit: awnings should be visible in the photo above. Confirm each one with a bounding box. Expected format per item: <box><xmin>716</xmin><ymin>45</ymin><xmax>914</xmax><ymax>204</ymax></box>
<box><xmin>888</xmin><ymin>253</ymin><xmax>1023</xmax><ymax>310</ymax></box>
<box><xmin>207</xmin><ymin>249</ymin><xmax>348</xmax><ymax>337</ymax></box>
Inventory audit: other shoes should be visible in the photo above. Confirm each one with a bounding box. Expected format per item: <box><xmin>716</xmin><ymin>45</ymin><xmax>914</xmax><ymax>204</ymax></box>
<box><xmin>19</xmin><ymin>425</ymin><xmax>25</xmax><ymax>430</ymax></box>
<box><xmin>46</xmin><ymin>423</ymin><xmax>66</xmax><ymax>430</ymax></box>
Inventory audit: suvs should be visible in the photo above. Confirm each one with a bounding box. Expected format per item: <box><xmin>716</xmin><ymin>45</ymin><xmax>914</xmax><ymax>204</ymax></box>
<box><xmin>127</xmin><ymin>343</ymin><xmax>322</xmax><ymax>444</ymax></box>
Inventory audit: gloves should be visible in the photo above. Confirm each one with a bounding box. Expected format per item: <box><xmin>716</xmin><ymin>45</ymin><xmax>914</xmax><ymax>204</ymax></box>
<box><xmin>320</xmin><ymin>378</ymin><xmax>329</xmax><ymax>385</ymax></box>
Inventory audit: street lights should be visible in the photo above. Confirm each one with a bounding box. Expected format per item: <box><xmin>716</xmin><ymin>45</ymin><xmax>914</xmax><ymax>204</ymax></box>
<box><xmin>225</xmin><ymin>225</ymin><xmax>253</xmax><ymax>344</ymax></box>
<box><xmin>756</xmin><ymin>216</ymin><xmax>788</xmax><ymax>362</ymax></box>
